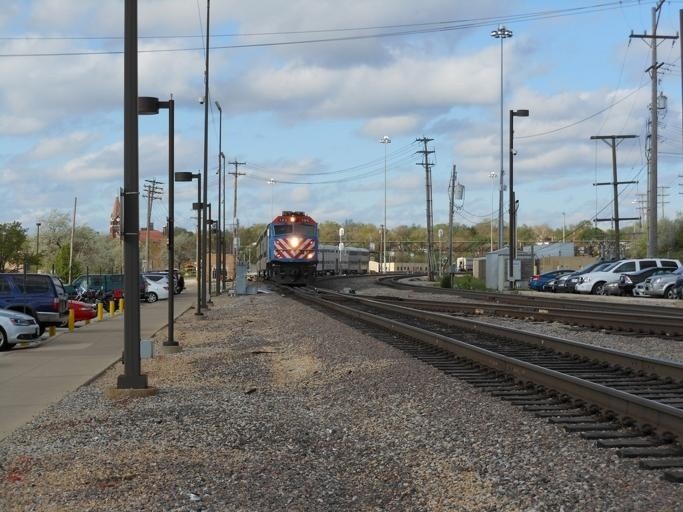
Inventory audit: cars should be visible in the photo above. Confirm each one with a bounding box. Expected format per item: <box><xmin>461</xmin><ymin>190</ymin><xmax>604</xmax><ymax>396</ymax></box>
<box><xmin>0</xmin><ymin>269</ymin><xmax>183</xmax><ymax>352</ymax></box>
<box><xmin>530</xmin><ymin>256</ymin><xmax>681</xmax><ymax>301</ymax></box>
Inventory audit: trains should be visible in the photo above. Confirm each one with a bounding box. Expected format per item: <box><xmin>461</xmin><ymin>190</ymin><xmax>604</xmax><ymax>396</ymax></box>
<box><xmin>250</xmin><ymin>210</ymin><xmax>372</xmax><ymax>284</ymax></box>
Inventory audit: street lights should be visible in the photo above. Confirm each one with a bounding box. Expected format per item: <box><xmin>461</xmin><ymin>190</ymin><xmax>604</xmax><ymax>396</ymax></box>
<box><xmin>507</xmin><ymin>107</ymin><xmax>530</xmax><ymax>294</ymax></box>
<box><xmin>267</xmin><ymin>176</ymin><xmax>277</xmax><ymax>220</ymax></box>
<box><xmin>33</xmin><ymin>222</ymin><xmax>42</xmax><ymax>275</ymax></box>
<box><xmin>176</xmin><ymin>167</ymin><xmax>229</xmax><ymax>316</ymax></box>
<box><xmin>631</xmin><ymin>199</ymin><xmax>638</xmax><ymax>235</ymax></box>
<box><xmin>377</xmin><ymin>133</ymin><xmax>391</xmax><ymax>273</ymax></box>
<box><xmin>487</xmin><ymin>170</ymin><xmax>496</xmax><ymax>251</ymax></box>
<box><xmin>135</xmin><ymin>92</ymin><xmax>183</xmax><ymax>356</ymax></box>
<box><xmin>561</xmin><ymin>210</ymin><xmax>568</xmax><ymax>243</ymax></box>
<box><xmin>489</xmin><ymin>19</ymin><xmax>517</xmax><ymax>296</ymax></box>
